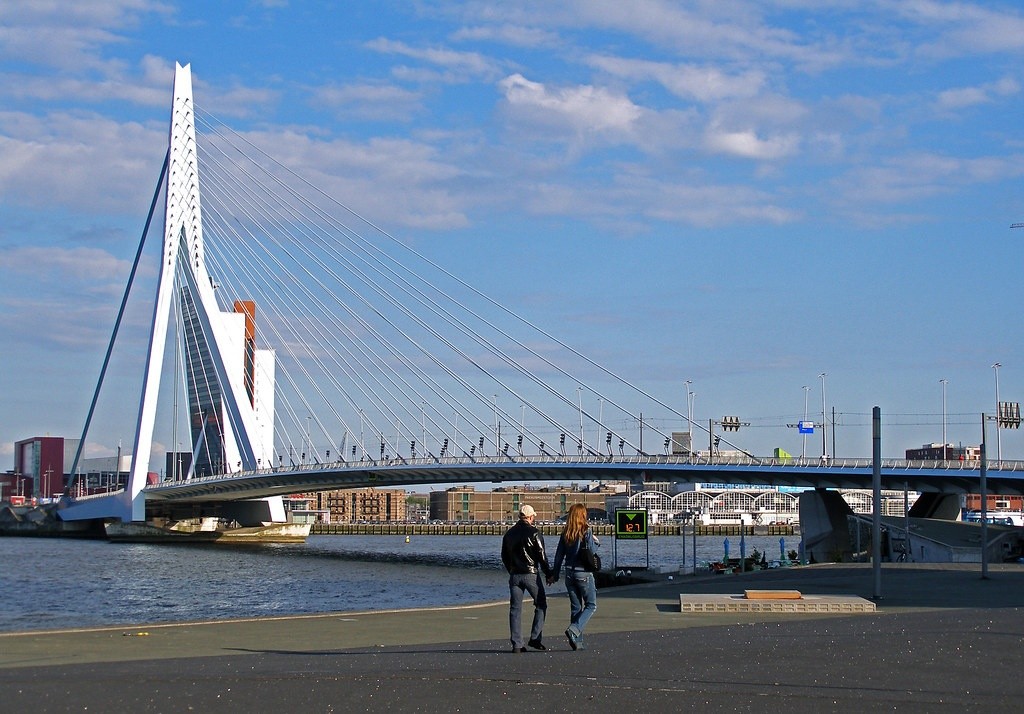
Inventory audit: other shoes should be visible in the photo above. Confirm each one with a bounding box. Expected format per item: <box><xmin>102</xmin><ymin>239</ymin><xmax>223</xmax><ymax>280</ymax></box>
<box><xmin>528</xmin><ymin>639</ymin><xmax>546</xmax><ymax>650</ymax></box>
<box><xmin>575</xmin><ymin>642</ymin><xmax>587</xmax><ymax>650</ymax></box>
<box><xmin>565</xmin><ymin>628</ymin><xmax>577</xmax><ymax>650</ymax></box>
<box><xmin>513</xmin><ymin>646</ymin><xmax>526</xmax><ymax>652</ymax></box>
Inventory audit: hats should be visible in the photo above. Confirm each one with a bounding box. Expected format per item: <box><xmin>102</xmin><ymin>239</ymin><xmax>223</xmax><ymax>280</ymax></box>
<box><xmin>518</xmin><ymin>505</ymin><xmax>537</xmax><ymax>518</ymax></box>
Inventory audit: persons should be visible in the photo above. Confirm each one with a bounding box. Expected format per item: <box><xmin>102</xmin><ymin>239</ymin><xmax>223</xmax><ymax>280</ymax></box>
<box><xmin>549</xmin><ymin>504</ymin><xmax>600</xmax><ymax>649</ymax></box>
<box><xmin>501</xmin><ymin>505</ymin><xmax>552</xmax><ymax>653</ymax></box>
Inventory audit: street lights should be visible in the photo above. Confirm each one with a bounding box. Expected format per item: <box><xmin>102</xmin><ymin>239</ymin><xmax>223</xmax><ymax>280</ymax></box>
<box><xmin>818</xmin><ymin>373</ymin><xmax>829</xmax><ymax>456</ymax></box>
<box><xmin>598</xmin><ymin>399</ymin><xmax>605</xmax><ymax>453</ymax></box>
<box><xmin>14</xmin><ymin>470</ymin><xmax>113</xmax><ymax>499</ymax></box>
<box><xmin>492</xmin><ymin>394</ymin><xmax>500</xmax><ymax>455</ymax></box>
<box><xmin>684</xmin><ymin>380</ymin><xmax>695</xmax><ymax>460</ymax></box>
<box><xmin>991</xmin><ymin>362</ymin><xmax>1002</xmax><ymax>468</ymax></box>
<box><xmin>939</xmin><ymin>379</ymin><xmax>949</xmax><ymax>469</ymax></box>
<box><xmin>305</xmin><ymin>417</ymin><xmax>312</xmax><ymax>462</ymax></box>
<box><xmin>360</xmin><ymin>409</ymin><xmax>365</xmax><ymax>462</ymax></box>
<box><xmin>396</xmin><ymin>420</ymin><xmax>401</xmax><ymax>458</ymax></box>
<box><xmin>421</xmin><ymin>402</ymin><xmax>427</xmax><ymax>457</ymax></box>
<box><xmin>519</xmin><ymin>405</ymin><xmax>527</xmax><ymax>456</ymax></box>
<box><xmin>178</xmin><ymin>443</ymin><xmax>182</xmax><ymax>481</ymax></box>
<box><xmin>576</xmin><ymin>387</ymin><xmax>583</xmax><ymax>454</ymax></box>
<box><xmin>452</xmin><ymin>413</ymin><xmax>459</xmax><ymax>456</ymax></box>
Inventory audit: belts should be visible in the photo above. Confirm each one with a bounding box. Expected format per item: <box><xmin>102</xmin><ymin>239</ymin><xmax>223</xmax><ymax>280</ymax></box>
<box><xmin>565</xmin><ymin>566</ymin><xmax>584</xmax><ymax>571</ymax></box>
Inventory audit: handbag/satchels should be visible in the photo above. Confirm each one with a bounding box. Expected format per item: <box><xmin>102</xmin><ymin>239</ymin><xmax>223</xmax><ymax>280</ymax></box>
<box><xmin>577</xmin><ymin>534</ymin><xmax>601</xmax><ymax>572</ymax></box>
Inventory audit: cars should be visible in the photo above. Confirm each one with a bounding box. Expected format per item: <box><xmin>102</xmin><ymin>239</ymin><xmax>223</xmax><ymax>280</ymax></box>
<box><xmin>352</xmin><ymin>520</ymin><xmax>568</xmax><ymax>525</ymax></box>
